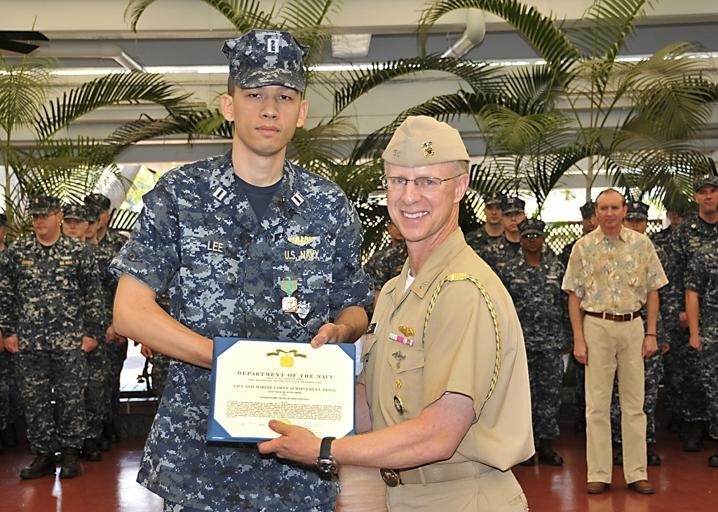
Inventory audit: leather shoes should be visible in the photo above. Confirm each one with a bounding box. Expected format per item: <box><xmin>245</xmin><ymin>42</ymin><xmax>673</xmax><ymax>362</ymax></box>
<box><xmin>628</xmin><ymin>480</ymin><xmax>656</xmax><ymax>495</ymax></box>
<box><xmin>585</xmin><ymin>481</ymin><xmax>610</xmax><ymax>494</ymax></box>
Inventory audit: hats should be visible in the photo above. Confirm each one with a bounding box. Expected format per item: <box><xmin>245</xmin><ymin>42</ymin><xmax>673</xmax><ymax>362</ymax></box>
<box><xmin>221</xmin><ymin>28</ymin><xmax>311</xmax><ymax>93</ymax></box>
<box><xmin>482</xmin><ymin>173</ymin><xmax>718</xmax><ymax>239</ymax></box>
<box><xmin>381</xmin><ymin>115</ymin><xmax>470</xmax><ymax>168</ymax></box>
<box><xmin>0</xmin><ymin>193</ymin><xmax>111</xmax><ymax>225</ymax></box>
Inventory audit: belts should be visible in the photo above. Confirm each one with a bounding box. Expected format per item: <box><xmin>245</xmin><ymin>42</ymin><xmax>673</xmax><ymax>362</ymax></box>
<box><xmin>584</xmin><ymin>309</ymin><xmax>642</xmax><ymax>322</ymax></box>
<box><xmin>379</xmin><ymin>460</ymin><xmax>494</xmax><ymax>488</ymax></box>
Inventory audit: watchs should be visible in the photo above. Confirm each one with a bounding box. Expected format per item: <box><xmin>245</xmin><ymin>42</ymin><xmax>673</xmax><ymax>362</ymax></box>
<box><xmin>316</xmin><ymin>437</ymin><xmax>338</xmax><ymax>476</ymax></box>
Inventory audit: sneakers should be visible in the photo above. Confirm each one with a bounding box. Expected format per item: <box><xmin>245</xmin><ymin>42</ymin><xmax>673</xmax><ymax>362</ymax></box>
<box><xmin>59</xmin><ymin>447</ymin><xmax>82</xmax><ymax>480</ymax></box>
<box><xmin>20</xmin><ymin>451</ymin><xmax>57</xmax><ymax>481</ymax></box>
<box><xmin>0</xmin><ymin>420</ymin><xmax>119</xmax><ymax>461</ymax></box>
<box><xmin>520</xmin><ymin>456</ymin><xmax>535</xmax><ymax>468</ymax></box>
<box><xmin>539</xmin><ymin>438</ymin><xmax>564</xmax><ymax>466</ymax></box>
<box><xmin>606</xmin><ymin>419</ymin><xmax>715</xmax><ymax>469</ymax></box>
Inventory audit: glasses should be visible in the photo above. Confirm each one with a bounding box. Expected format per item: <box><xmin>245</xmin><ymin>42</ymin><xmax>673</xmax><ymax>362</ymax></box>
<box><xmin>379</xmin><ymin>172</ymin><xmax>467</xmax><ymax>192</ymax></box>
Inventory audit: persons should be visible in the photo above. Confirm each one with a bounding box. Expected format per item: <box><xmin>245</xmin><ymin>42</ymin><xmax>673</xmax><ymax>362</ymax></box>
<box><xmin>256</xmin><ymin>114</ymin><xmax>537</xmax><ymax>511</ymax></box>
<box><xmin>611</xmin><ymin>174</ymin><xmax>717</xmax><ymax>467</ymax></box>
<box><xmin>108</xmin><ymin>30</ymin><xmax>375</xmax><ymax>510</ymax></box>
<box><xmin>464</xmin><ymin>189</ymin><xmax>596</xmax><ymax>468</ymax></box>
<box><xmin>561</xmin><ymin>188</ymin><xmax>669</xmax><ymax>495</ymax></box>
<box><xmin>362</xmin><ymin>217</ymin><xmax>408</xmax><ymax>298</ymax></box>
<box><xmin>0</xmin><ymin>193</ymin><xmax>169</xmax><ymax>481</ymax></box>
